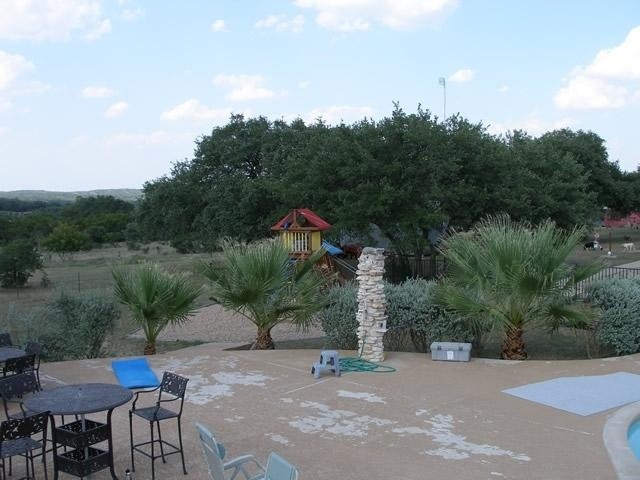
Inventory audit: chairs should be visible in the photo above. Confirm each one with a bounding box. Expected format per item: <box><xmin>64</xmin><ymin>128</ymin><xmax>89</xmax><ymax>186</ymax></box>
<box><xmin>0</xmin><ymin>331</ymin><xmax>189</xmax><ymax>480</ymax></box>
<box><xmin>195</xmin><ymin>423</ymin><xmax>299</xmax><ymax>480</ymax></box>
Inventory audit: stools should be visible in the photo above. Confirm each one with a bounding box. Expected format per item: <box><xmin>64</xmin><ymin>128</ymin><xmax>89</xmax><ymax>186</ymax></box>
<box><xmin>309</xmin><ymin>351</ymin><xmax>341</xmax><ymax>379</ymax></box>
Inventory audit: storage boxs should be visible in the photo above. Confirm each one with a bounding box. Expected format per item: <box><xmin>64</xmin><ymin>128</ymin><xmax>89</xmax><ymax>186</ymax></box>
<box><xmin>429</xmin><ymin>342</ymin><xmax>473</xmax><ymax>362</ymax></box>
